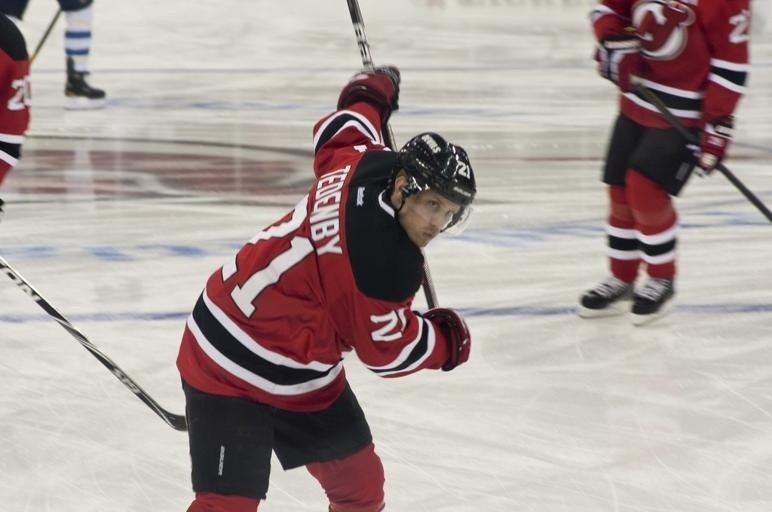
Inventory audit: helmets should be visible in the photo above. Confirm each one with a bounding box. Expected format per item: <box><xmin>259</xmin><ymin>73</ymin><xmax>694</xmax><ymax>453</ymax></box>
<box><xmin>394</xmin><ymin>132</ymin><xmax>475</xmax><ymax>234</ymax></box>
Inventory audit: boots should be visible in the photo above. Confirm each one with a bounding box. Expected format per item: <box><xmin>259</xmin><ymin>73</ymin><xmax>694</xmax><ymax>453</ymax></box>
<box><xmin>64</xmin><ymin>59</ymin><xmax>104</xmax><ymax>98</ymax></box>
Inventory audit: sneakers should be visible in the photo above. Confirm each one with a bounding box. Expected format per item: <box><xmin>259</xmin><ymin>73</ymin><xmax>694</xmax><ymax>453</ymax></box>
<box><xmin>582</xmin><ymin>275</ymin><xmax>634</xmax><ymax>310</ymax></box>
<box><xmin>634</xmin><ymin>277</ymin><xmax>673</xmax><ymax>315</ymax></box>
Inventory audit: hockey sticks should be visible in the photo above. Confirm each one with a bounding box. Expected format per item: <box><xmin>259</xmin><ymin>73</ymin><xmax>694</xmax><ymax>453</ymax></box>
<box><xmin>0</xmin><ymin>256</ymin><xmax>187</xmax><ymax>429</ymax></box>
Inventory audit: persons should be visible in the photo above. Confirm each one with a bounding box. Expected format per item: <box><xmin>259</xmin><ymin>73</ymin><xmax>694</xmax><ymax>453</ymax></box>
<box><xmin>175</xmin><ymin>66</ymin><xmax>476</xmax><ymax>512</ymax></box>
<box><xmin>0</xmin><ymin>0</ymin><xmax>105</xmax><ymax>100</ymax></box>
<box><xmin>578</xmin><ymin>0</ymin><xmax>751</xmax><ymax>316</ymax></box>
<box><xmin>0</xmin><ymin>13</ymin><xmax>31</xmax><ymax>215</ymax></box>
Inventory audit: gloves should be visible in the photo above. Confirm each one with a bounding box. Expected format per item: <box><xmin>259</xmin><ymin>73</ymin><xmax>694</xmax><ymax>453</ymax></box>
<box><xmin>422</xmin><ymin>308</ymin><xmax>469</xmax><ymax>370</ymax></box>
<box><xmin>336</xmin><ymin>66</ymin><xmax>400</xmax><ymax>116</ymax></box>
<box><xmin>695</xmin><ymin>118</ymin><xmax>733</xmax><ymax>178</ymax></box>
<box><xmin>598</xmin><ymin>38</ymin><xmax>637</xmax><ymax>92</ymax></box>
